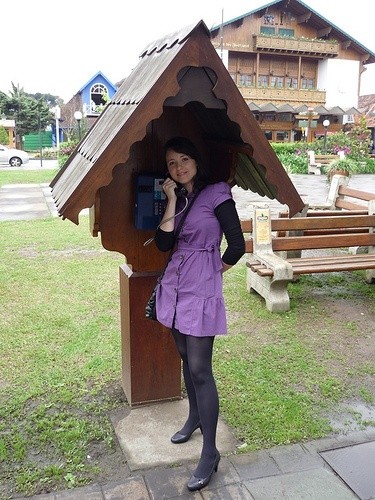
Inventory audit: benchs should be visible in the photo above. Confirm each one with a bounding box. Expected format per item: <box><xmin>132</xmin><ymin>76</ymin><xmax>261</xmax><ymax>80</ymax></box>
<box><xmin>240</xmin><ymin>175</ymin><xmax>375</xmax><ymax>314</ymax></box>
<box><xmin>308</xmin><ymin>151</ymin><xmax>345</xmax><ymax>175</ymax></box>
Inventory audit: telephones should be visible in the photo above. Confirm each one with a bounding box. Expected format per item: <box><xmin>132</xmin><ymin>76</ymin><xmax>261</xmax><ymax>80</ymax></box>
<box><xmin>159</xmin><ymin>160</ymin><xmax>189</xmax><ymax>200</ymax></box>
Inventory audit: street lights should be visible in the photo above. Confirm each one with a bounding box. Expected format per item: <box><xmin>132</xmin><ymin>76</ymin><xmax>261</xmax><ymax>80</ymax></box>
<box><xmin>74</xmin><ymin>110</ymin><xmax>83</xmax><ymax>141</ymax></box>
<box><xmin>322</xmin><ymin>120</ymin><xmax>331</xmax><ymax>152</ymax></box>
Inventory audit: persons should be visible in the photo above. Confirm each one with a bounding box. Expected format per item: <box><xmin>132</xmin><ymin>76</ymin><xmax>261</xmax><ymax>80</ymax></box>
<box><xmin>155</xmin><ymin>140</ymin><xmax>247</xmax><ymax>491</ymax></box>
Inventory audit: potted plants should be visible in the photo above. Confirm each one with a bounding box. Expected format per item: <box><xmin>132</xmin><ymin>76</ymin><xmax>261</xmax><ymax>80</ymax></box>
<box><xmin>326</xmin><ymin>159</ymin><xmax>355</xmax><ymax>181</ymax></box>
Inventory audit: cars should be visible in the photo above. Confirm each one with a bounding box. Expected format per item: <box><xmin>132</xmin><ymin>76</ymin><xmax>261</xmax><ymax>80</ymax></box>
<box><xmin>0</xmin><ymin>142</ymin><xmax>30</xmax><ymax>168</ymax></box>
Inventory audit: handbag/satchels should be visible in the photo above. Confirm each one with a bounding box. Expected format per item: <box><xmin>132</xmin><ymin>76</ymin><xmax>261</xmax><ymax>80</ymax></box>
<box><xmin>145</xmin><ymin>284</ymin><xmax>157</xmax><ymax>322</ymax></box>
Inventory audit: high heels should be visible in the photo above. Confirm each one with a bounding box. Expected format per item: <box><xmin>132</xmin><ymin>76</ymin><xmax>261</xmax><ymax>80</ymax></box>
<box><xmin>187</xmin><ymin>448</ymin><xmax>220</xmax><ymax>492</ymax></box>
<box><xmin>171</xmin><ymin>423</ymin><xmax>203</xmax><ymax>444</ymax></box>
